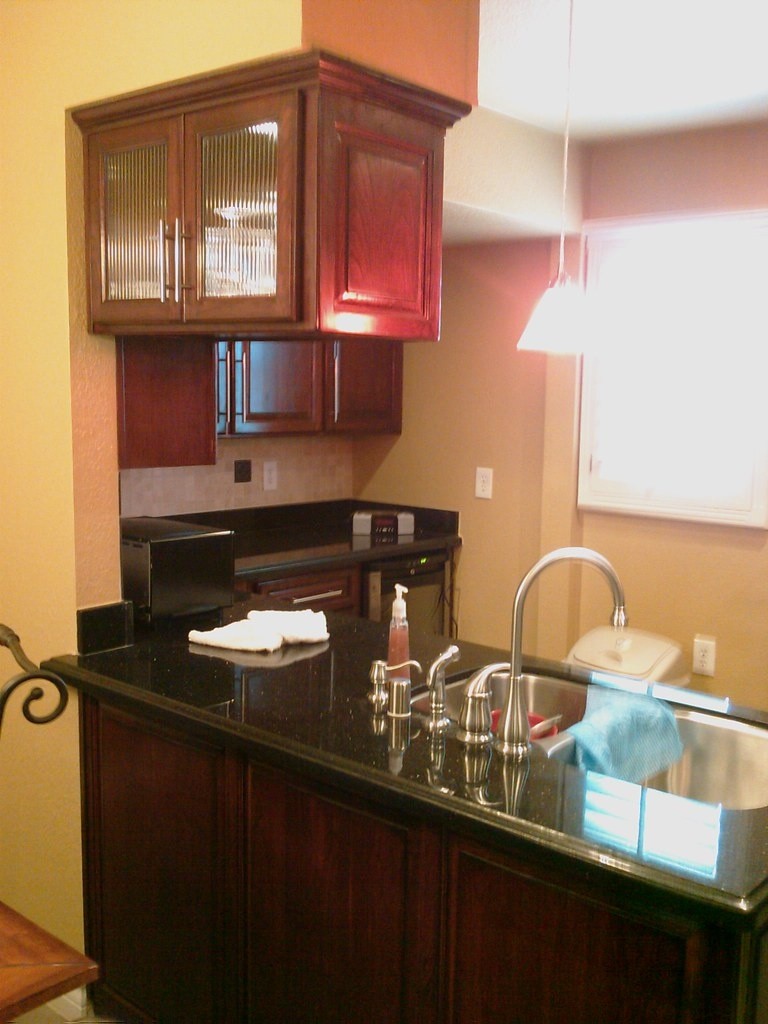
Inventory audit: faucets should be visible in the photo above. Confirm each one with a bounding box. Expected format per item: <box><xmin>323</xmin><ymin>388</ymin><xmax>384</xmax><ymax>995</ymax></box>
<box><xmin>495</xmin><ymin>544</ymin><xmax>628</xmax><ymax>739</ymax></box>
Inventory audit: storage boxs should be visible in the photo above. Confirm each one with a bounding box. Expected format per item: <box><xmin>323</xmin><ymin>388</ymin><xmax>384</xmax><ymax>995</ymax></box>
<box><xmin>352</xmin><ymin>511</ymin><xmax>414</xmax><ymax>536</ymax></box>
<box><xmin>352</xmin><ymin>534</ymin><xmax>414</xmax><ymax>554</ymax></box>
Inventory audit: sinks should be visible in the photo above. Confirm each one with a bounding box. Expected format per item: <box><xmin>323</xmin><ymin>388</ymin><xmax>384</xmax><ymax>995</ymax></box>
<box><xmin>550</xmin><ymin>715</ymin><xmax>768</xmax><ymax>810</ymax></box>
<box><xmin>411</xmin><ymin>670</ymin><xmax>613</xmax><ymax>743</ymax></box>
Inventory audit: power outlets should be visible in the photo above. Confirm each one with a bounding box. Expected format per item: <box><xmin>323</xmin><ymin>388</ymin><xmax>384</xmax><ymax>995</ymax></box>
<box><xmin>263</xmin><ymin>462</ymin><xmax>277</xmax><ymax>490</ymax></box>
<box><xmin>235</xmin><ymin>460</ymin><xmax>251</xmax><ymax>483</ymax></box>
<box><xmin>692</xmin><ymin>636</ymin><xmax>717</xmax><ymax>677</ymax></box>
<box><xmin>475</xmin><ymin>467</ymin><xmax>493</xmax><ymax>498</ymax></box>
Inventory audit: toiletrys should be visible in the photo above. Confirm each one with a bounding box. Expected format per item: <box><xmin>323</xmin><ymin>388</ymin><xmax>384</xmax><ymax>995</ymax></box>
<box><xmin>387</xmin><ymin>583</ymin><xmax>411</xmax><ymax>676</ymax></box>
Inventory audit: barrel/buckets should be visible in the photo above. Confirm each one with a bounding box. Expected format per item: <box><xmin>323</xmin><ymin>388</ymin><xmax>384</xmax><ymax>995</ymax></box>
<box><xmin>489</xmin><ymin>708</ymin><xmax>558</xmax><ymax>745</ymax></box>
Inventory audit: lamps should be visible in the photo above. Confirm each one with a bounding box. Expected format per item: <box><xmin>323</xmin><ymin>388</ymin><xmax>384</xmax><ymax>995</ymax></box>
<box><xmin>518</xmin><ymin>2</ymin><xmax>619</xmax><ymax>360</ymax></box>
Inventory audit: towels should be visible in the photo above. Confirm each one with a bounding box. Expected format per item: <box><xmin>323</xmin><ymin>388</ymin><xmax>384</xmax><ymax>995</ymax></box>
<box><xmin>247</xmin><ymin>609</ymin><xmax>330</xmax><ymax>644</ymax></box>
<box><xmin>188</xmin><ymin>618</ymin><xmax>284</xmax><ymax>652</ymax></box>
<box><xmin>185</xmin><ymin>639</ymin><xmax>331</xmax><ymax>667</ymax></box>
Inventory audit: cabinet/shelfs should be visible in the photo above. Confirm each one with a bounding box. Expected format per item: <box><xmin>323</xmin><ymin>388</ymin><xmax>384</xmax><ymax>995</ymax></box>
<box><xmin>72</xmin><ymin>48</ymin><xmax>446</xmax><ymax>342</ymax></box>
<box><xmin>257</xmin><ymin>566</ymin><xmax>364</xmax><ymax>615</ymax></box>
<box><xmin>31</xmin><ymin>615</ymin><xmax>768</xmax><ymax>1024</ymax></box>
<box><xmin>117</xmin><ymin>338</ymin><xmax>403</xmax><ymax>469</ymax></box>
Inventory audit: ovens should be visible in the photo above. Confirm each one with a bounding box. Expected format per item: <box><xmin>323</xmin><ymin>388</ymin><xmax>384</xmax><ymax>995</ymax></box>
<box><xmin>365</xmin><ymin>554</ymin><xmax>452</xmax><ymax>637</ymax></box>
